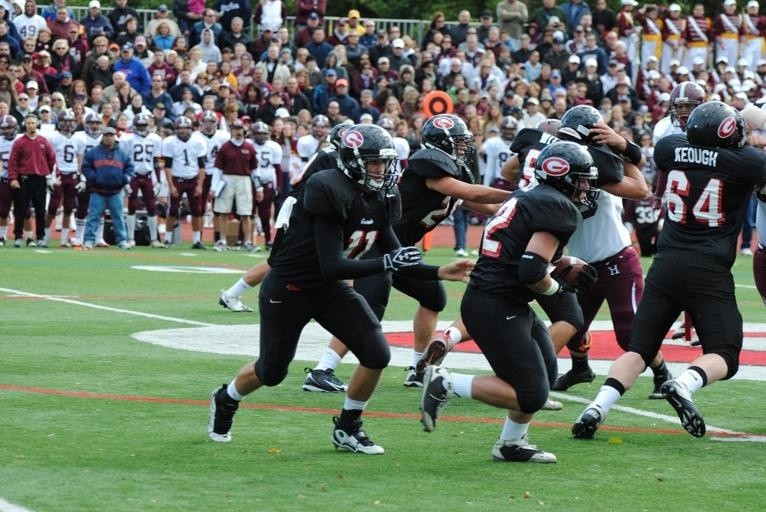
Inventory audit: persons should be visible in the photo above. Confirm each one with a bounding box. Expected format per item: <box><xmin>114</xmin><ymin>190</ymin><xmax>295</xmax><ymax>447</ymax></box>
<box><xmin>0</xmin><ymin>0</ymin><xmax>766</xmax><ymax>253</ymax></box>
<box><xmin>198</xmin><ymin>82</ymin><xmax>766</xmax><ymax>463</ymax></box>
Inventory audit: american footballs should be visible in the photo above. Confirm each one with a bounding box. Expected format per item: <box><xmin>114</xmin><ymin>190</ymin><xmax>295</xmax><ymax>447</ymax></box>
<box><xmin>549</xmin><ymin>256</ymin><xmax>587</xmax><ymax>283</ymax></box>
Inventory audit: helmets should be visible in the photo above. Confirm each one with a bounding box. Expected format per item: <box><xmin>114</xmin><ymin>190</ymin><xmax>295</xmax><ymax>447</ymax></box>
<box><xmin>669</xmin><ymin>81</ymin><xmax>706</xmax><ymax>127</ymax></box>
<box><xmin>336</xmin><ymin>124</ymin><xmax>399</xmax><ymax>196</ymax></box>
<box><xmin>687</xmin><ymin>101</ymin><xmax>743</xmax><ymax>150</ymax></box>
<box><xmin>534</xmin><ymin>140</ymin><xmax>599</xmax><ymax>220</ymax></box>
<box><xmin>557</xmin><ymin>104</ymin><xmax>602</xmax><ymax>145</ymax></box>
<box><xmin>419</xmin><ymin>115</ymin><xmax>476</xmax><ymax>166</ymax></box>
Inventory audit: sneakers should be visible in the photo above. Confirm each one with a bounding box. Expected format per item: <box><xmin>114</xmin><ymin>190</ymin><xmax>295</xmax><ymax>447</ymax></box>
<box><xmin>455</xmin><ymin>248</ymin><xmax>468</xmax><ymax>257</ymax></box>
<box><xmin>0</xmin><ymin>222</ymin><xmax>273</xmax><ymax>252</ymax></box>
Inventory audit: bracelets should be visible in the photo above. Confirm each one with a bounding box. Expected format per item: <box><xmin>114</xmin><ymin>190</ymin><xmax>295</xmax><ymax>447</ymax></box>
<box><xmin>541</xmin><ymin>275</ymin><xmax>560</xmax><ymax>296</ymax></box>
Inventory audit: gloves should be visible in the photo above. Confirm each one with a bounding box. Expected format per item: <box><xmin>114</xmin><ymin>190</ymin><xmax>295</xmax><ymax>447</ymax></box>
<box><xmin>550</xmin><ymin>264</ymin><xmax>599</xmax><ymax>293</ymax></box>
<box><xmin>383</xmin><ymin>245</ymin><xmax>422</xmax><ymax>273</ymax></box>
